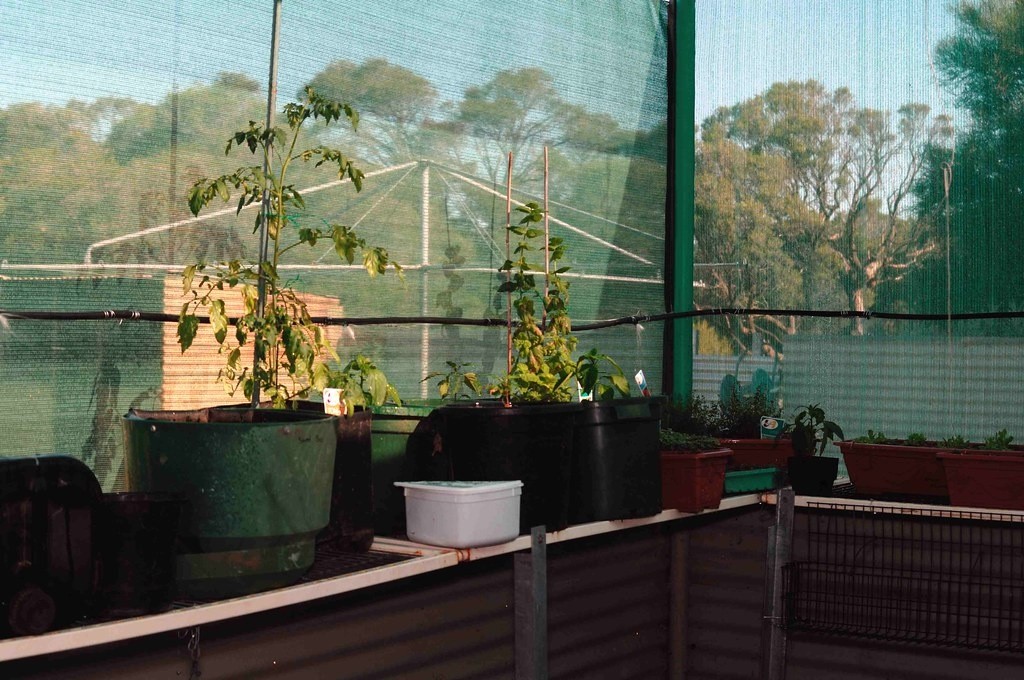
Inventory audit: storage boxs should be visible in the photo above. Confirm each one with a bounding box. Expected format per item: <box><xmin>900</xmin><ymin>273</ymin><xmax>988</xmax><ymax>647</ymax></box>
<box><xmin>392</xmin><ymin>478</ymin><xmax>524</xmax><ymax>549</ymax></box>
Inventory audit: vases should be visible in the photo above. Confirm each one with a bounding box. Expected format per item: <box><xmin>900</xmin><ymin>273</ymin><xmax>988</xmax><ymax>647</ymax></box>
<box><xmin>100</xmin><ymin>491</ymin><xmax>178</xmax><ymax>616</ymax></box>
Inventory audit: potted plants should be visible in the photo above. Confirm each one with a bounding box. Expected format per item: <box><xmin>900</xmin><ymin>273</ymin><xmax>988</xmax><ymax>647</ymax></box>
<box><xmin>180</xmin><ymin>85</ymin><xmax>408</xmax><ymax>552</ymax></box>
<box><xmin>418</xmin><ymin>201</ymin><xmax>586</xmax><ymax>536</ymax></box>
<box><xmin>658</xmin><ymin>379</ymin><xmax>839</xmax><ymax>513</ymax></box>
<box><xmin>834</xmin><ymin>428</ymin><xmax>1024</xmax><ymax>512</ymax></box>
<box><xmin>574</xmin><ymin>346</ymin><xmax>668</xmax><ymax>522</ymax></box>
